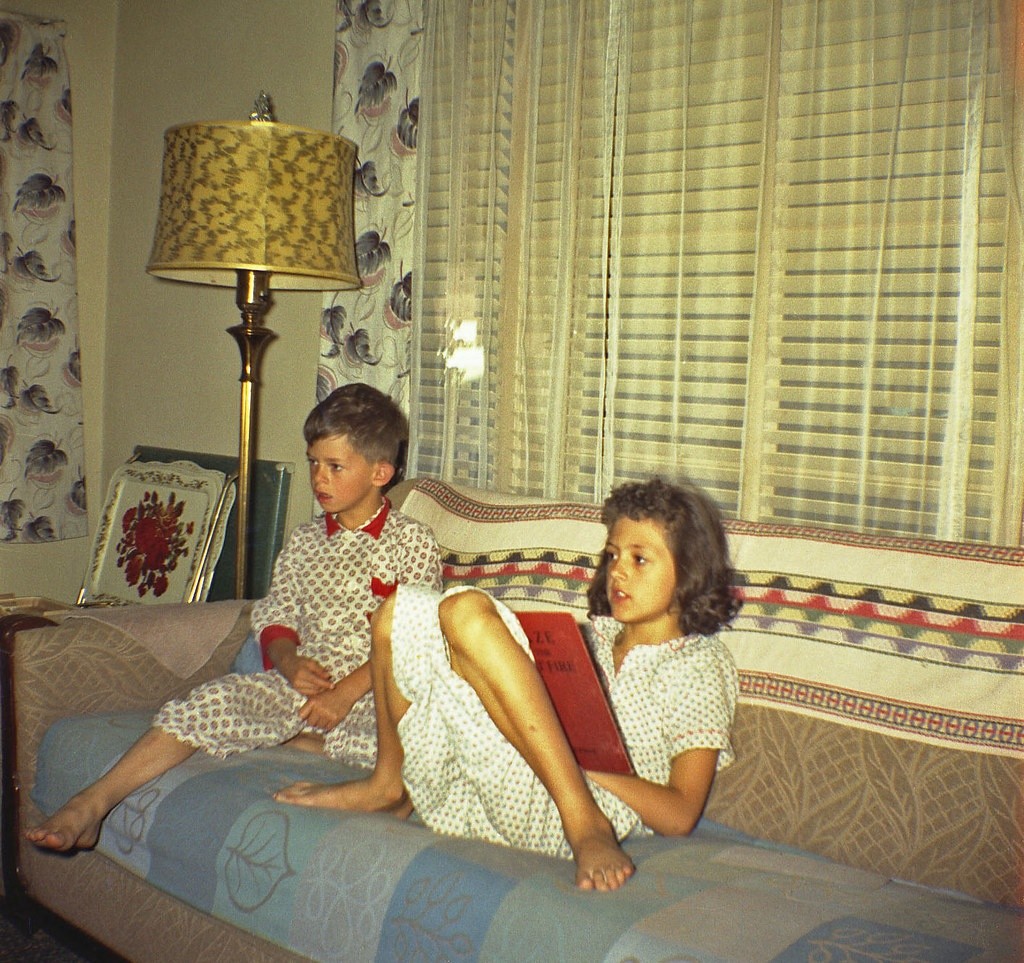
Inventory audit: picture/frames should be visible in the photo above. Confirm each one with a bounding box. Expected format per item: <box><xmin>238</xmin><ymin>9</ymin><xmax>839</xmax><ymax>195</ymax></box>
<box><xmin>74</xmin><ymin>460</ymin><xmax>237</xmax><ymax>613</ymax></box>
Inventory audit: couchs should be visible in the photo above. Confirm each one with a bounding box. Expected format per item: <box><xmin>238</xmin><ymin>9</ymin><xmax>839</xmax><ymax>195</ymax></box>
<box><xmin>12</xmin><ymin>477</ymin><xmax>1024</xmax><ymax>963</ymax></box>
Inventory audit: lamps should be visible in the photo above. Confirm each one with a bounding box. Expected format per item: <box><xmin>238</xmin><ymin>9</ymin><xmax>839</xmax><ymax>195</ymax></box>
<box><xmin>144</xmin><ymin>90</ymin><xmax>363</xmax><ymax>598</ymax></box>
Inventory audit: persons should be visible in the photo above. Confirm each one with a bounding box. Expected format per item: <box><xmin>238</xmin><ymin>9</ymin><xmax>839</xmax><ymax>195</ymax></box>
<box><xmin>22</xmin><ymin>381</ymin><xmax>443</xmax><ymax>853</ymax></box>
<box><xmin>272</xmin><ymin>478</ymin><xmax>744</xmax><ymax>892</ymax></box>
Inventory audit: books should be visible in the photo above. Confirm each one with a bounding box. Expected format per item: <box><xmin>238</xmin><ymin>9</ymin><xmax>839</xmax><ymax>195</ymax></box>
<box><xmin>514</xmin><ymin>608</ymin><xmax>634</xmax><ymax>777</ymax></box>
